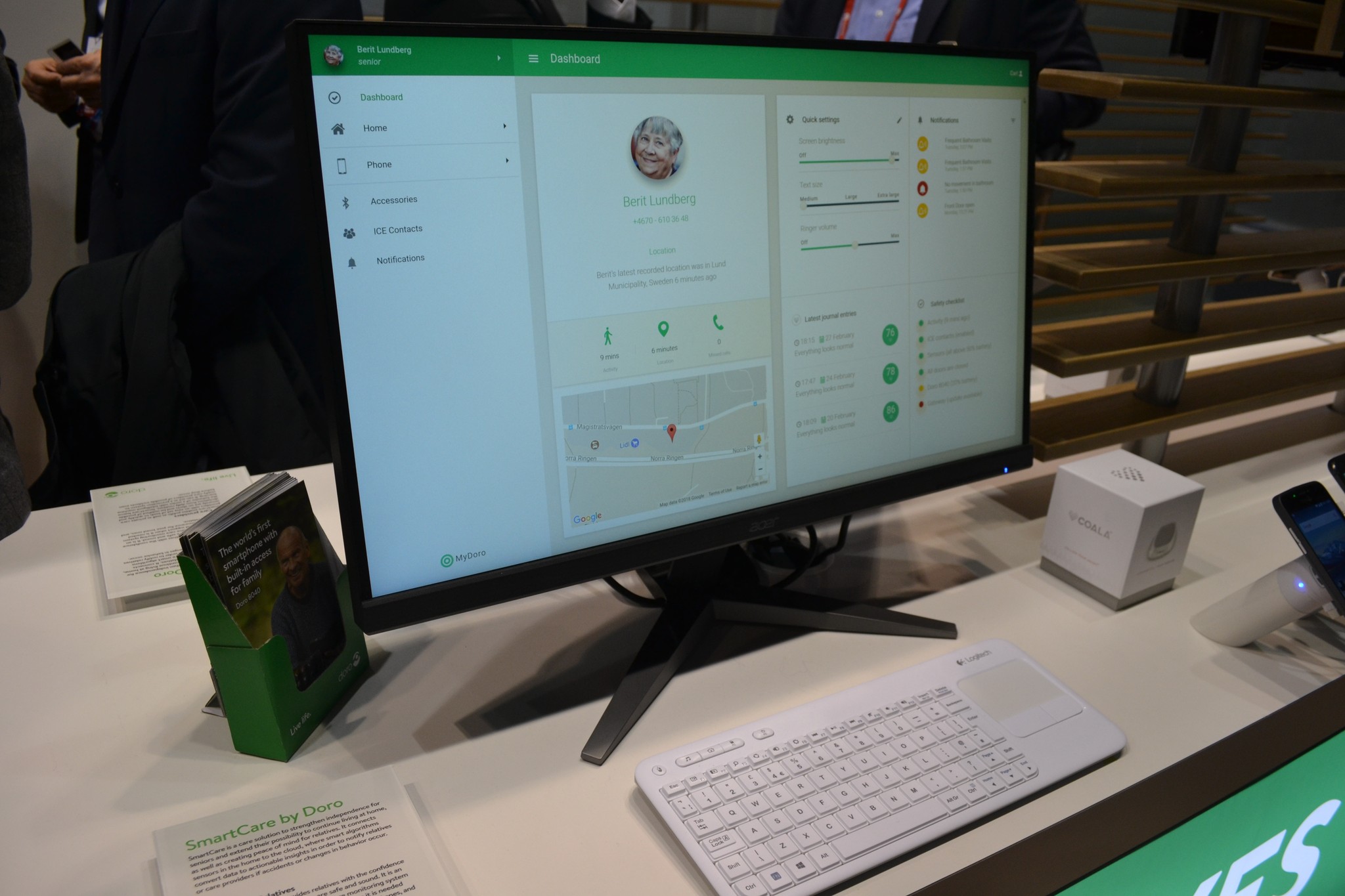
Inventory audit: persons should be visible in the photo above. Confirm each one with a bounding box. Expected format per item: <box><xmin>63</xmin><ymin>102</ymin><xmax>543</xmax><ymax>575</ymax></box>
<box><xmin>769</xmin><ymin>0</ymin><xmax>1112</xmax><ymax>171</ymax></box>
<box><xmin>20</xmin><ymin>0</ymin><xmax>370</xmax><ymax>515</ymax></box>
<box><xmin>631</xmin><ymin>115</ymin><xmax>682</xmax><ymax>181</ymax></box>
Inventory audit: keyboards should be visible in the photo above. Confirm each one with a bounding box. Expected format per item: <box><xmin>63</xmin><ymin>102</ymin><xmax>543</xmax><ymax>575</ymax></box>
<box><xmin>634</xmin><ymin>639</ymin><xmax>1129</xmax><ymax>896</ymax></box>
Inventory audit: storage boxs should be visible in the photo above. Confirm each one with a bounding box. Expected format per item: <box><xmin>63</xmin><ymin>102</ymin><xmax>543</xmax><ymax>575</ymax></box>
<box><xmin>1035</xmin><ymin>446</ymin><xmax>1208</xmax><ymax>615</ymax></box>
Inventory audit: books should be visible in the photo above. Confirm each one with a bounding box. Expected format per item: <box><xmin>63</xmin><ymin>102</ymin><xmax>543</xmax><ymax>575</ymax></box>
<box><xmin>178</xmin><ymin>472</ymin><xmax>350</xmax><ymax>704</ymax></box>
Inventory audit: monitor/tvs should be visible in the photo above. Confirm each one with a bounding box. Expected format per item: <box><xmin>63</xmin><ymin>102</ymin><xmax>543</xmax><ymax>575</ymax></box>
<box><xmin>286</xmin><ymin>19</ymin><xmax>1037</xmax><ymax>647</ymax></box>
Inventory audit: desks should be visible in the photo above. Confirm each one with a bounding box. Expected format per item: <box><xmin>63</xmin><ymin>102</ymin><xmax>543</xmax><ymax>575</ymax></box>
<box><xmin>0</xmin><ymin>382</ymin><xmax>1344</xmax><ymax>896</ymax></box>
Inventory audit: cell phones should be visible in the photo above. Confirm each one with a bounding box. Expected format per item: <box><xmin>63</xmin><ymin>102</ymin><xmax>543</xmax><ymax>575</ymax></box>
<box><xmin>1271</xmin><ymin>480</ymin><xmax>1344</xmax><ymax>620</ymax></box>
